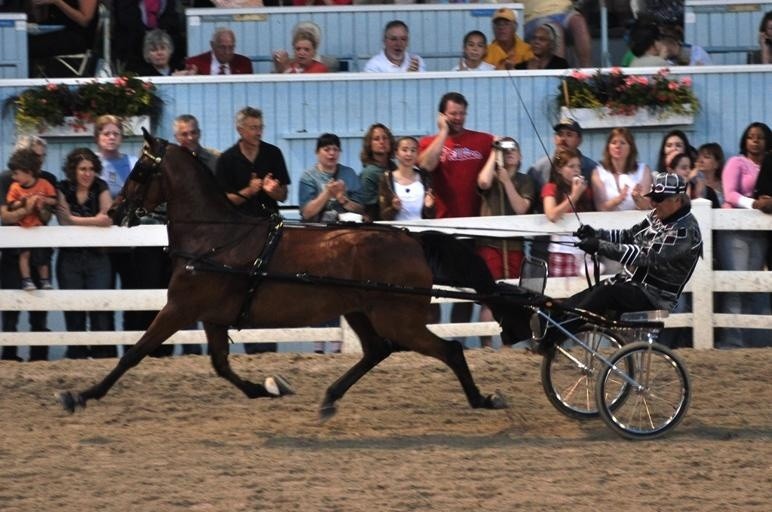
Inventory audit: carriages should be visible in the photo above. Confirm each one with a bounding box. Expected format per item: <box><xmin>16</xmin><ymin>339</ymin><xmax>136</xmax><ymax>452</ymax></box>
<box><xmin>53</xmin><ymin>125</ymin><xmax>705</xmax><ymax>443</ymax></box>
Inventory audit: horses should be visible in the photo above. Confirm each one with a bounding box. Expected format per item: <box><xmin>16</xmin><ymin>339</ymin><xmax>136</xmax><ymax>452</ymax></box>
<box><xmin>52</xmin><ymin>126</ymin><xmax>510</xmax><ymax>427</ymax></box>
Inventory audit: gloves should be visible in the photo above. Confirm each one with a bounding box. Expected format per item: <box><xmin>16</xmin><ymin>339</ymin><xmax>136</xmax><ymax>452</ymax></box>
<box><xmin>577</xmin><ymin>225</ymin><xmax>600</xmax><ymax>254</ymax></box>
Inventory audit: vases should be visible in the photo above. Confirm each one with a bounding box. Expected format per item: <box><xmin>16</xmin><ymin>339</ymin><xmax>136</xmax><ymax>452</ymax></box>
<box><xmin>19</xmin><ymin>114</ymin><xmax>150</xmax><ymax>142</ymax></box>
<box><xmin>557</xmin><ymin>104</ymin><xmax>694</xmax><ymax>131</ymax></box>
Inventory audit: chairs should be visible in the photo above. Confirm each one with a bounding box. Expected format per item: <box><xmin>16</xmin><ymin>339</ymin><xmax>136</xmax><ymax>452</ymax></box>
<box><xmin>611</xmin><ymin>240</ymin><xmax>703</xmax><ymax>346</ymax></box>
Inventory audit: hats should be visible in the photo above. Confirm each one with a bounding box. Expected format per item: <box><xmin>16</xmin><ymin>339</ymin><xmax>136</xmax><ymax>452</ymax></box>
<box><xmin>554</xmin><ymin>119</ymin><xmax>581</xmax><ymax>132</ymax></box>
<box><xmin>492</xmin><ymin>9</ymin><xmax>516</xmax><ymax>22</ymax></box>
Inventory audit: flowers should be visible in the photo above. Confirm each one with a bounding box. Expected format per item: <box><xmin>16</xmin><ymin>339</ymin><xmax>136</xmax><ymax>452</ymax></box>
<box><xmin>2</xmin><ymin>71</ymin><xmax>166</xmax><ymax>132</ymax></box>
<box><xmin>547</xmin><ymin>68</ymin><xmax>709</xmax><ymax>116</ymax></box>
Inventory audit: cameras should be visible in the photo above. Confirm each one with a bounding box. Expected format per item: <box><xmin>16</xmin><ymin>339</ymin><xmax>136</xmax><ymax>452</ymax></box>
<box><xmin>492</xmin><ymin>138</ymin><xmax>515</xmax><ymax>150</ymax></box>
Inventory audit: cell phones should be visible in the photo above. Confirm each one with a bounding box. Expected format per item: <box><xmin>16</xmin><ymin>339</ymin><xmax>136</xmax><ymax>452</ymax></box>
<box><xmin>449</xmin><ymin>123</ymin><xmax>455</xmax><ymax>133</ymax></box>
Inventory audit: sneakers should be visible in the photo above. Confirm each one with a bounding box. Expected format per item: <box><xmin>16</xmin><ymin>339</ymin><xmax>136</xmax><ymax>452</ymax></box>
<box><xmin>39</xmin><ymin>279</ymin><xmax>52</xmax><ymax>289</ymax></box>
<box><xmin>22</xmin><ymin>277</ymin><xmax>36</xmax><ymax>289</ymax></box>
<box><xmin>643</xmin><ymin>173</ymin><xmax>686</xmax><ymax>199</ymax></box>
<box><xmin>529</xmin><ymin>313</ymin><xmax>556</xmax><ymax>355</ymax></box>
